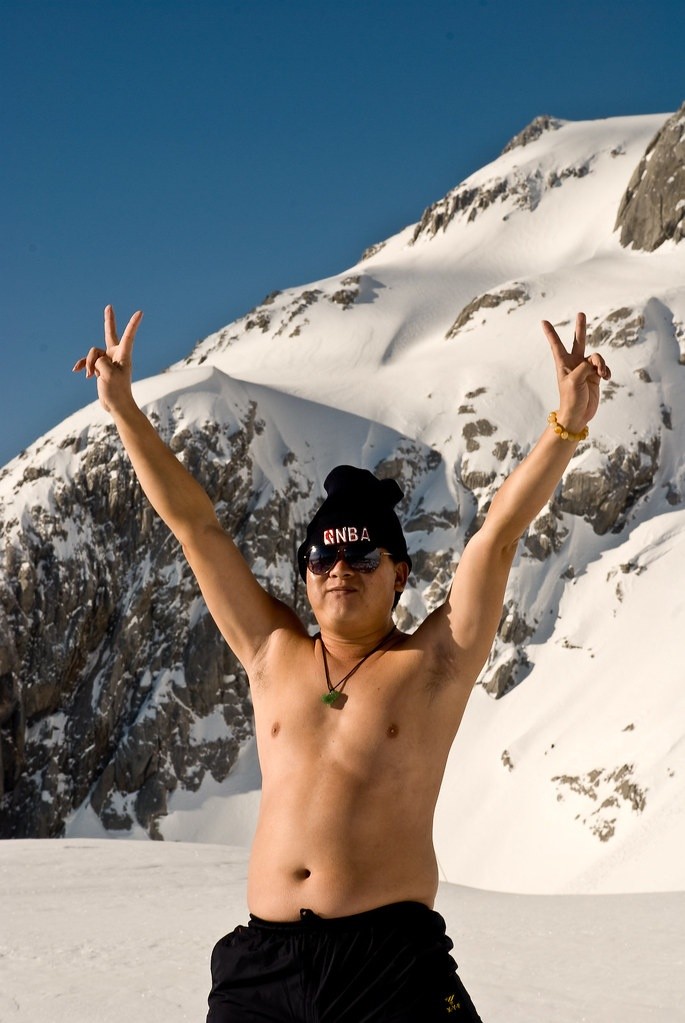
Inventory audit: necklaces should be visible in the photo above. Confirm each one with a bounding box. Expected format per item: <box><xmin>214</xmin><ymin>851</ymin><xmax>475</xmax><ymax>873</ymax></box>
<box><xmin>321</xmin><ymin>626</ymin><xmax>397</xmax><ymax>704</ymax></box>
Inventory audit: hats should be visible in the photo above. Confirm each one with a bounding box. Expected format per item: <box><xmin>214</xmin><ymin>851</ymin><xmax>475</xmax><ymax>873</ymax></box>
<box><xmin>298</xmin><ymin>465</ymin><xmax>412</xmax><ymax>582</ymax></box>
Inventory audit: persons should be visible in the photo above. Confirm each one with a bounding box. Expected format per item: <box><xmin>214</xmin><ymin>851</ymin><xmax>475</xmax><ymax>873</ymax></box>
<box><xmin>72</xmin><ymin>305</ymin><xmax>612</xmax><ymax>1023</ymax></box>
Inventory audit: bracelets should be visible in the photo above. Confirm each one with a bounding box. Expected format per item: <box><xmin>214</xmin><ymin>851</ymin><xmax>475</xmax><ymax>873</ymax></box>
<box><xmin>548</xmin><ymin>410</ymin><xmax>589</xmax><ymax>441</ymax></box>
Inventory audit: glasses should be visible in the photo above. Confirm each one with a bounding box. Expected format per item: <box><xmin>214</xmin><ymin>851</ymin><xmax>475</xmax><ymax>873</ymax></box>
<box><xmin>302</xmin><ymin>541</ymin><xmax>392</xmax><ymax>575</ymax></box>
